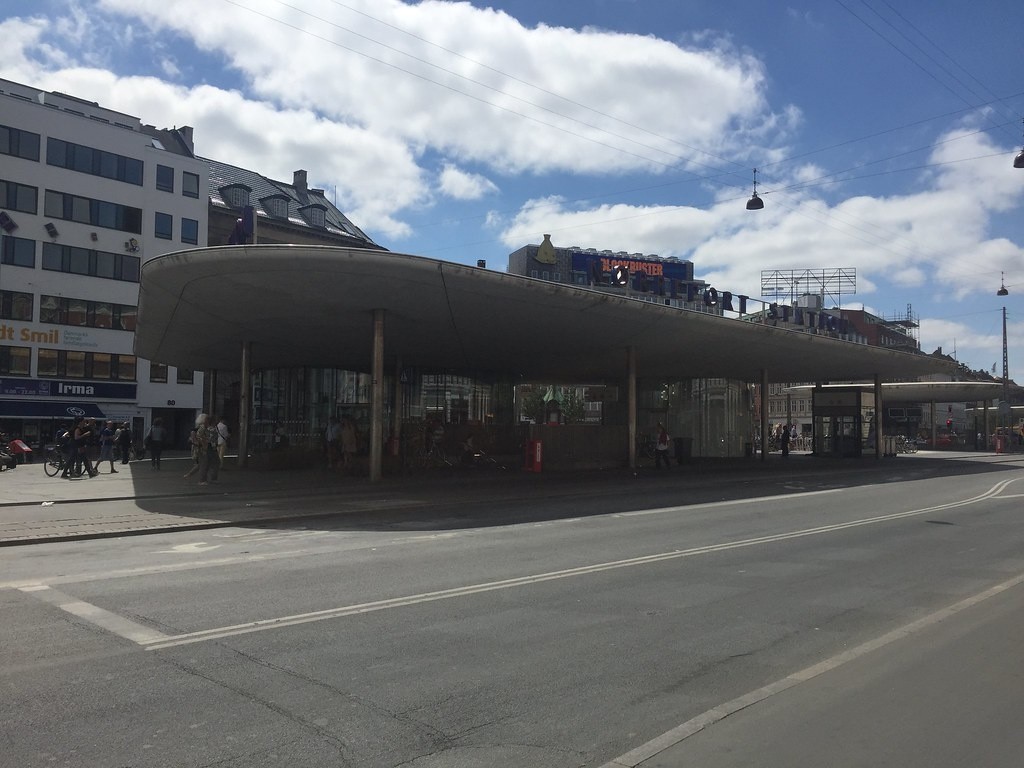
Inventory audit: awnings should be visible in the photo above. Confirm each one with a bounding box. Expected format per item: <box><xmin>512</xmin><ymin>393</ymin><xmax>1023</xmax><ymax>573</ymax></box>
<box><xmin>0</xmin><ymin>400</ymin><xmax>108</xmax><ymax>420</ymax></box>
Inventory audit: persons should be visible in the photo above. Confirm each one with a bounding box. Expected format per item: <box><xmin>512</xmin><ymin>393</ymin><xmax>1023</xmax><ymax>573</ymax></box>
<box><xmin>61</xmin><ymin>416</ymin><xmax>99</xmax><ymax>479</ymax></box>
<box><xmin>464</xmin><ymin>434</ymin><xmax>475</xmax><ymax>454</ymax></box>
<box><xmin>781</xmin><ymin>426</ymin><xmax>790</xmax><ymax>456</ymax></box>
<box><xmin>274</xmin><ymin>421</ymin><xmax>287</xmax><ymax>457</ymax></box>
<box><xmin>844</xmin><ymin>424</ymin><xmax>856</xmax><ymax>436</ymax></box>
<box><xmin>183</xmin><ymin>414</ymin><xmax>231</xmax><ymax>484</ymax></box>
<box><xmin>147</xmin><ymin>417</ymin><xmax>165</xmax><ymax>471</ymax></box>
<box><xmin>426</xmin><ymin>413</ymin><xmax>445</xmax><ymax>457</ymax></box>
<box><xmin>655</xmin><ymin>424</ymin><xmax>671</xmax><ymax>471</ymax></box>
<box><xmin>977</xmin><ymin>432</ymin><xmax>985</xmax><ymax>445</ymax></box>
<box><xmin>113</xmin><ymin>421</ymin><xmax>131</xmax><ymax>464</ymax></box>
<box><xmin>0</xmin><ymin>432</ymin><xmax>18</xmax><ymax>456</ymax></box>
<box><xmin>768</xmin><ymin>423</ymin><xmax>808</xmax><ymax>451</ymax></box>
<box><xmin>324</xmin><ymin>414</ymin><xmax>357</xmax><ymax>471</ymax></box>
<box><xmin>94</xmin><ymin>421</ymin><xmax>119</xmax><ymax>473</ymax></box>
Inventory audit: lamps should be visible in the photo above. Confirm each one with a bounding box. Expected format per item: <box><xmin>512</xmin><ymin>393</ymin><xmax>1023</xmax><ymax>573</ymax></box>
<box><xmin>1013</xmin><ymin>148</ymin><xmax>1024</xmax><ymax>168</ymax></box>
<box><xmin>747</xmin><ymin>170</ymin><xmax>764</xmax><ymax>209</ymax></box>
<box><xmin>997</xmin><ymin>271</ymin><xmax>1008</xmax><ymax>295</ymax></box>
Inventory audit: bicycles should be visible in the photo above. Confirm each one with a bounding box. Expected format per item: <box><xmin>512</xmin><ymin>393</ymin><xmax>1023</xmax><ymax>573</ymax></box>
<box><xmin>136</xmin><ymin>444</ymin><xmax>152</xmax><ymax>460</ymax></box>
<box><xmin>896</xmin><ymin>436</ymin><xmax>919</xmax><ymax>455</ymax></box>
<box><xmin>44</xmin><ymin>444</ymin><xmax>87</xmax><ymax>477</ymax></box>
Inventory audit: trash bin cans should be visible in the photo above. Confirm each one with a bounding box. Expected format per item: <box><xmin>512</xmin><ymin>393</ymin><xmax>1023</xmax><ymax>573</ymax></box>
<box><xmin>673</xmin><ymin>437</ymin><xmax>692</xmax><ymax>464</ymax></box>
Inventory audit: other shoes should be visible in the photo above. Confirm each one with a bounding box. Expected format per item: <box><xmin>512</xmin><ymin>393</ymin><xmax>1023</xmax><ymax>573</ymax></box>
<box><xmin>61</xmin><ymin>474</ymin><xmax>68</xmax><ymax>478</ymax></box>
<box><xmin>90</xmin><ymin>473</ymin><xmax>97</xmax><ymax>478</ymax></box>
<box><xmin>201</xmin><ymin>481</ymin><xmax>208</xmax><ymax>486</ymax></box>
<box><xmin>111</xmin><ymin>469</ymin><xmax>118</xmax><ymax>473</ymax></box>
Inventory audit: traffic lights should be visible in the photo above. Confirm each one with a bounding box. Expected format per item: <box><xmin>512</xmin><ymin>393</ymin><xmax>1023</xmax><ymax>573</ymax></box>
<box><xmin>946</xmin><ymin>419</ymin><xmax>951</xmax><ymax>426</ymax></box>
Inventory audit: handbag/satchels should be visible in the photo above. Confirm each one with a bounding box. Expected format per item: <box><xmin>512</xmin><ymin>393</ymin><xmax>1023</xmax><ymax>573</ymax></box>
<box><xmin>331</xmin><ymin>439</ymin><xmax>339</xmax><ymax>444</ymax></box>
<box><xmin>144</xmin><ymin>431</ymin><xmax>161</xmax><ymax>450</ymax></box>
<box><xmin>226</xmin><ymin>438</ymin><xmax>231</xmax><ymax>447</ymax></box>
